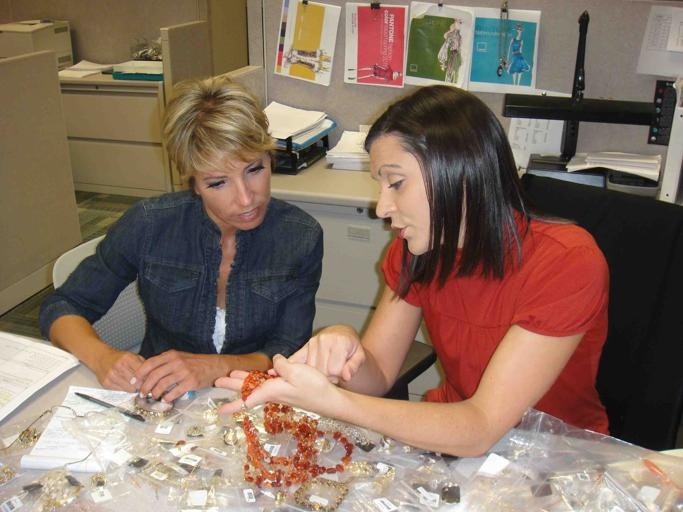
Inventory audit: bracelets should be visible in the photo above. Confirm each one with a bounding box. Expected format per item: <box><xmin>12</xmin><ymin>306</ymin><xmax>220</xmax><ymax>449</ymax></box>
<box><xmin>135</xmin><ymin>394</ymin><xmax>175</xmax><ymax>416</ymax></box>
<box><xmin>294</xmin><ymin>478</ymin><xmax>348</xmax><ymax>512</ymax></box>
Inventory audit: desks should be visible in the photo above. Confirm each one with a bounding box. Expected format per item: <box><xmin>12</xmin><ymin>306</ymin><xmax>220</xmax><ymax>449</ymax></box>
<box><xmin>1</xmin><ymin>332</ymin><xmax>680</xmax><ymax>511</ymax></box>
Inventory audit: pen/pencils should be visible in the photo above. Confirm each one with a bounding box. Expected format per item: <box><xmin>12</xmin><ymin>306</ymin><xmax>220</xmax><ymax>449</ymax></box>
<box><xmin>74</xmin><ymin>392</ymin><xmax>145</xmax><ymax>422</ymax></box>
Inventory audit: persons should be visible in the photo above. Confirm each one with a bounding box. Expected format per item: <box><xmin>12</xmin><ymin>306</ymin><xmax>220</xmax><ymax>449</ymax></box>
<box><xmin>282</xmin><ymin>47</ymin><xmax>332</xmax><ymax>73</ymax></box>
<box><xmin>438</xmin><ymin>19</ymin><xmax>462</xmax><ymax>83</ymax></box>
<box><xmin>506</xmin><ymin>25</ymin><xmax>530</xmax><ymax>85</ymax></box>
<box><xmin>348</xmin><ymin>63</ymin><xmax>403</xmax><ymax>81</ymax></box>
<box><xmin>214</xmin><ymin>84</ymin><xmax>611</xmax><ymax>458</ymax></box>
<box><xmin>38</xmin><ymin>73</ymin><xmax>324</xmax><ymax>404</ymax></box>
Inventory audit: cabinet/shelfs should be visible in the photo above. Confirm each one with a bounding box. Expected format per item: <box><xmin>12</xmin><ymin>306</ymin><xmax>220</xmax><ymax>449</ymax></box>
<box><xmin>270</xmin><ymin>150</ymin><xmax>446</xmax><ymax>402</ymax></box>
<box><xmin>60</xmin><ymin>31</ymin><xmax>171</xmax><ymax>197</ymax></box>
<box><xmin>1</xmin><ymin>48</ymin><xmax>84</xmax><ymax>318</ymax></box>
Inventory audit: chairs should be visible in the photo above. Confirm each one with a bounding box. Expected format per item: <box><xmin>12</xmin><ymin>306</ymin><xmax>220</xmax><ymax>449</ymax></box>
<box><xmin>381</xmin><ymin>173</ymin><xmax>681</xmax><ymax>451</ymax></box>
<box><xmin>53</xmin><ymin>233</ymin><xmax>147</xmax><ymax>357</ymax></box>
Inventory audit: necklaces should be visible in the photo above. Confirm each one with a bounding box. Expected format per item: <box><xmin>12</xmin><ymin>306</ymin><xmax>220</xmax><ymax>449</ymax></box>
<box><xmin>241</xmin><ymin>369</ymin><xmax>354</xmax><ymax>488</ymax></box>
<box><xmin>497</xmin><ymin>0</ymin><xmax>508</xmax><ymax>76</ymax></box>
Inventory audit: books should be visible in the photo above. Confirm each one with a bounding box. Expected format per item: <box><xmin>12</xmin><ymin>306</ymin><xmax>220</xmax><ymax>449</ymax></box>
<box><xmin>566</xmin><ymin>151</ymin><xmax>662</xmax><ymax>181</ymax></box>
<box><xmin>58</xmin><ymin>59</ymin><xmax>164</xmax><ymax>82</ymax></box>
<box><xmin>262</xmin><ymin>100</ymin><xmax>373</xmax><ymax>171</ymax></box>
